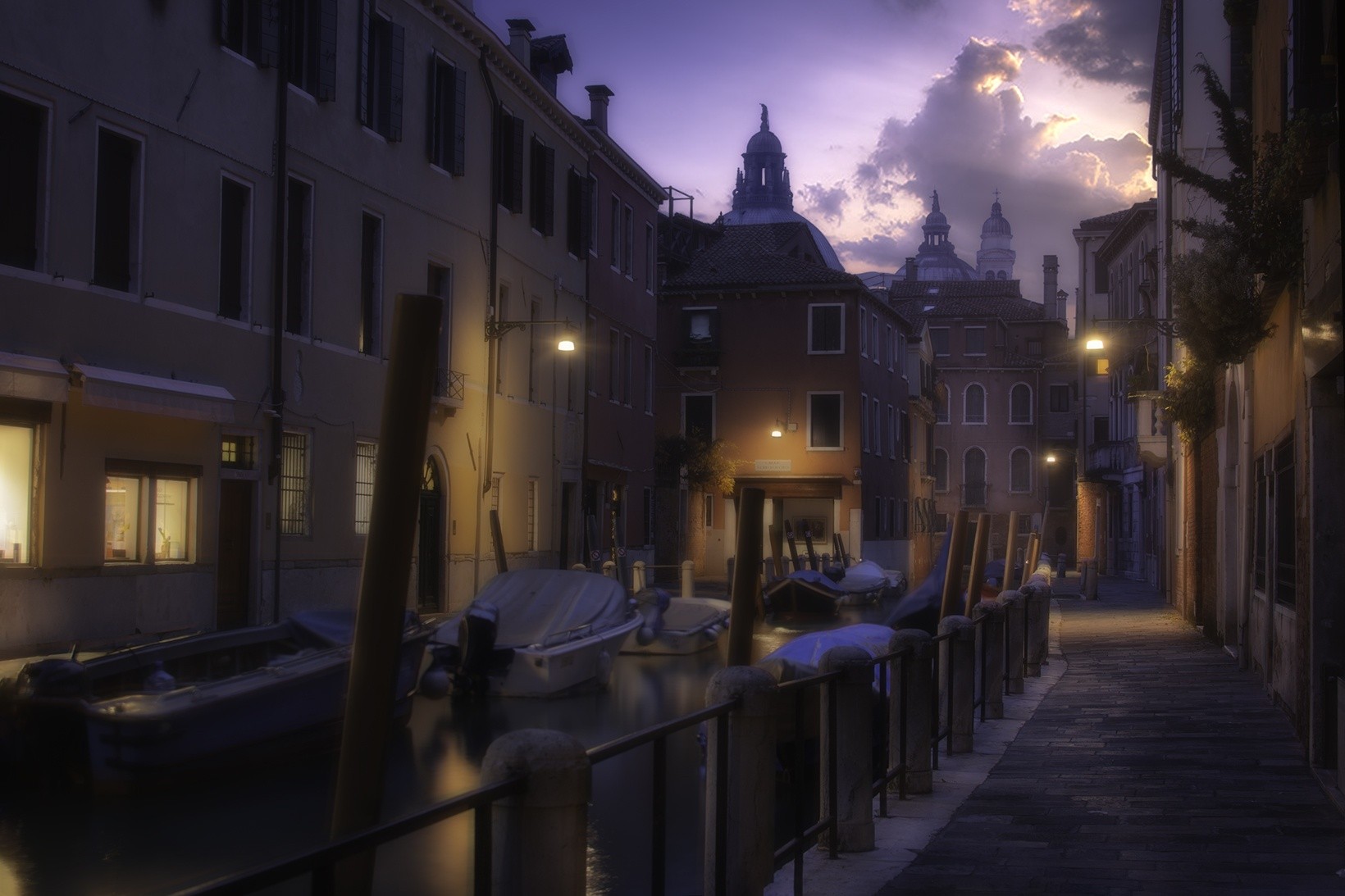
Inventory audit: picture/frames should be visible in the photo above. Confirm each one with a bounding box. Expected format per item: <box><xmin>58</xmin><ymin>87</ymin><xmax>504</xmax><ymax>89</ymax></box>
<box><xmin>794</xmin><ymin>515</ymin><xmax>829</xmax><ymax>544</ymax></box>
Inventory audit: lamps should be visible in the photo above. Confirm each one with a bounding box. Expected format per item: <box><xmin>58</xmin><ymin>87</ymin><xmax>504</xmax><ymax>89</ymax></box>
<box><xmin>772</xmin><ymin>419</ymin><xmax>798</xmax><ymax>437</ymax></box>
<box><xmin>484</xmin><ymin>320</ymin><xmax>579</xmax><ymax>352</ymax></box>
<box><xmin>1047</xmin><ymin>447</ymin><xmax>1078</xmax><ymax>463</ymax></box>
<box><xmin>1083</xmin><ymin>314</ymin><xmax>1181</xmax><ymax>355</ymax></box>
<box><xmin>58</xmin><ymin>356</ymin><xmax>85</xmax><ymax>391</ymax></box>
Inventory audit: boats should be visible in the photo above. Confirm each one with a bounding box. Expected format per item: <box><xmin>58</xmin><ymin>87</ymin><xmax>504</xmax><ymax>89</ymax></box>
<box><xmin>7</xmin><ymin>606</ymin><xmax>448</xmax><ymax>806</ymax></box>
<box><xmin>617</xmin><ymin>594</ymin><xmax>734</xmax><ymax>655</ymax></box>
<box><xmin>425</xmin><ymin>565</ymin><xmax>645</xmax><ymax>699</ymax></box>
<box><xmin>759</xmin><ymin>557</ymin><xmax>908</xmax><ymax>622</ymax></box>
<box><xmin>750</xmin><ymin>622</ymin><xmax>896</xmax><ymax>709</ymax></box>
